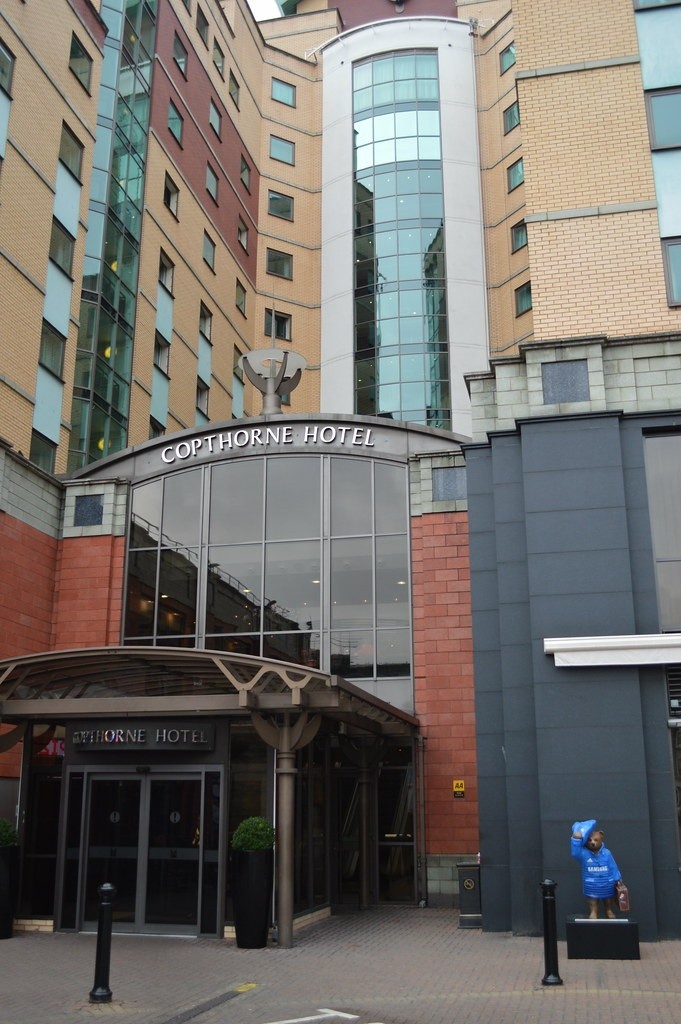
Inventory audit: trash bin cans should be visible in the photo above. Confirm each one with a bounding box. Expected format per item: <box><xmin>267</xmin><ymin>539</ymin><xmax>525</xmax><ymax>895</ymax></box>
<box><xmin>456</xmin><ymin>861</ymin><xmax>482</xmax><ymax>929</ymax></box>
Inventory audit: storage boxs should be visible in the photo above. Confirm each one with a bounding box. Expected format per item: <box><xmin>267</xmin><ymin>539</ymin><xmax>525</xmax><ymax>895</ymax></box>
<box><xmin>566</xmin><ymin>921</ymin><xmax>640</xmax><ymax>960</ymax></box>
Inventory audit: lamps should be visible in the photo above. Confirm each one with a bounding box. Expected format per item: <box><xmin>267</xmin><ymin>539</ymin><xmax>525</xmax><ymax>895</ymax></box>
<box><xmin>336</xmin><ymin>721</ymin><xmax>348</xmax><ymax>738</ymax></box>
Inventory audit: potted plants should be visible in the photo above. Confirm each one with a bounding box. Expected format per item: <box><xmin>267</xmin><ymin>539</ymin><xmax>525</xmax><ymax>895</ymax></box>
<box><xmin>230</xmin><ymin>815</ymin><xmax>277</xmax><ymax>950</ymax></box>
<box><xmin>0</xmin><ymin>817</ymin><xmax>21</xmax><ymax>939</ymax></box>
